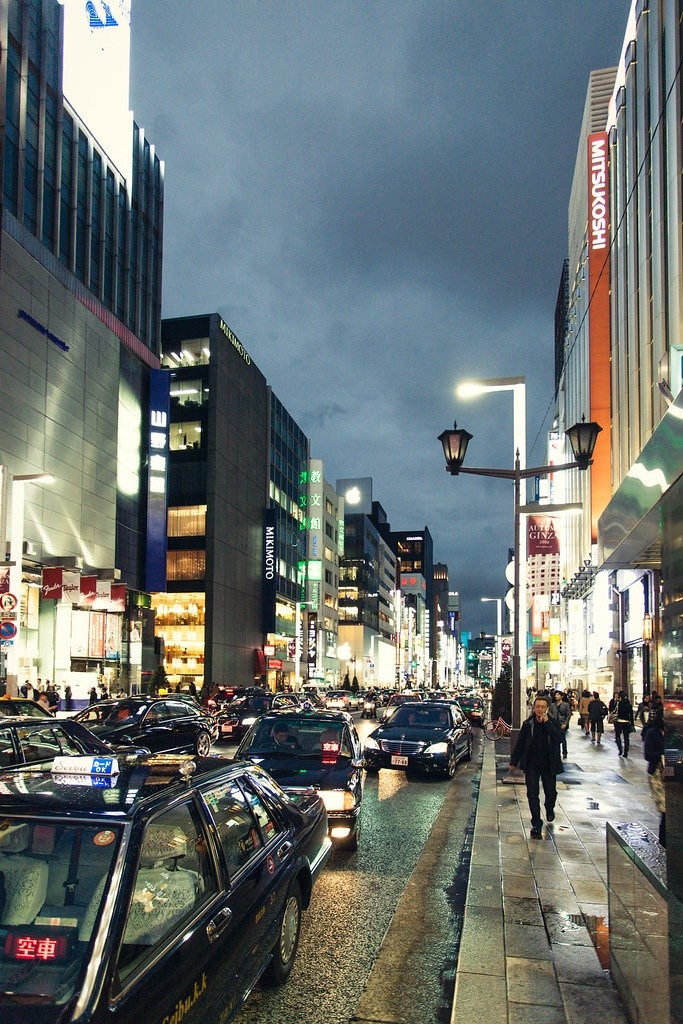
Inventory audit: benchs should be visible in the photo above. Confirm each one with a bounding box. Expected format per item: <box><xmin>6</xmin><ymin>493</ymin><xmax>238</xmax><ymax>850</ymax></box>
<box><xmin>297</xmin><ymin>732</ymin><xmax>320</xmax><ymax>752</ymax></box>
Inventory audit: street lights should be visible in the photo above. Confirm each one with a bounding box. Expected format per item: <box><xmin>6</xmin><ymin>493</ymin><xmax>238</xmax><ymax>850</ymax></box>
<box><xmin>295</xmin><ymin>601</ymin><xmax>313</xmax><ymax>691</ymax></box>
<box><xmin>451</xmin><ymin>376</ymin><xmax>531</xmax><ymax>734</ymax></box>
<box><xmin>370</xmin><ymin>634</ymin><xmax>383</xmax><ymax>688</ymax></box>
<box><xmin>438</xmin><ymin>412</ymin><xmax>602</xmax><ymax>786</ymax></box>
<box><xmin>481</xmin><ymin>596</ymin><xmax>503</xmax><ymax>686</ymax></box>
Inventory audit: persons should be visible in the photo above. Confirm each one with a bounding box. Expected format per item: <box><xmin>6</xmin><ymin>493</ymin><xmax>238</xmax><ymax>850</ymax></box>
<box><xmin>64</xmin><ymin>685</ymin><xmax>72</xmax><ymax>711</ymax></box>
<box><xmin>609</xmin><ymin>691</ymin><xmax>634</xmax><ymax>758</ymax></box>
<box><xmin>21</xmin><ymin>679</ymin><xmax>61</xmax><ymax>716</ymax></box>
<box><xmin>579</xmin><ymin>690</ymin><xmax>593</xmax><ymax>735</ymax></box>
<box><xmin>200</xmin><ymin>682</ymin><xmax>219</xmax><ymax>704</ymax></box>
<box><xmin>439</xmin><ymin>712</ymin><xmax>448</xmax><ymax>721</ymax></box>
<box><xmin>360</xmin><ymin>689</ymin><xmax>378</xmax><ymax>719</ymax></box>
<box><xmin>266</xmin><ymin>723</ymin><xmax>297</xmax><ymax>750</ymax></box>
<box><xmin>526</xmin><ymin>687</ymin><xmax>555</xmax><ymax>706</ymax></box>
<box><xmin>189</xmin><ymin>682</ymin><xmax>197</xmax><ymax>696</ymax></box>
<box><xmin>510</xmin><ymin>697</ymin><xmax>565</xmax><ymax>835</ymax></box>
<box><xmin>101</xmin><ymin>688</ymin><xmax>108</xmax><ymax>699</ymax></box>
<box><xmin>408</xmin><ymin>712</ymin><xmax>415</xmax><ymax>724</ymax></box>
<box><xmin>313</xmin><ymin>728</ymin><xmax>347</xmax><ymax>752</ymax></box>
<box><xmin>262</xmin><ymin>683</ymin><xmax>272</xmax><ymax>691</ymax></box>
<box><xmin>85</xmin><ymin>687</ymin><xmax>99</xmax><ymax>719</ymax></box>
<box><xmin>644</xmin><ymin>718</ymin><xmax>664</xmax><ymax>776</ymax></box>
<box><xmin>567</xmin><ymin>689</ymin><xmax>579</xmax><ymax>710</ymax></box>
<box><xmin>636</xmin><ymin>691</ymin><xmax>664</xmax><ymax>740</ymax></box>
<box><xmin>119</xmin><ymin>707</ymin><xmax>156</xmax><ymax>717</ymax></box>
<box><xmin>551</xmin><ymin>692</ymin><xmax>571</xmax><ymax>758</ymax></box>
<box><xmin>119</xmin><ymin>688</ymin><xmax>125</xmax><ymax>698</ymax></box>
<box><xmin>176</xmin><ymin>682</ymin><xmax>181</xmax><ymax>693</ymax></box>
<box><xmin>289</xmin><ymin>685</ymin><xmax>293</xmax><ymax>692</ymax></box>
<box><xmin>588</xmin><ymin>693</ymin><xmax>604</xmax><ymax>743</ymax></box>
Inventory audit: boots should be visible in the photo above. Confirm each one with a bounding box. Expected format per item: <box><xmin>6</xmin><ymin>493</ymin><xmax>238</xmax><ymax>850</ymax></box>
<box><xmin>591</xmin><ymin>732</ymin><xmax>595</xmax><ymax>741</ymax></box>
<box><xmin>597</xmin><ymin>733</ymin><xmax>601</xmax><ymax>743</ymax></box>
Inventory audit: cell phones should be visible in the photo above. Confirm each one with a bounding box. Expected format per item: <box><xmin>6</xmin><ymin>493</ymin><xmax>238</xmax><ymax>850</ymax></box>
<box><xmin>540</xmin><ymin>707</ymin><xmax>550</xmax><ymax>721</ymax></box>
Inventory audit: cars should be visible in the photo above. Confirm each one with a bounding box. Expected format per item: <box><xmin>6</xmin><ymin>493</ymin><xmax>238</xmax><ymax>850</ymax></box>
<box><xmin>231</xmin><ymin>706</ymin><xmax>365</xmax><ymax>850</ymax></box>
<box><xmin>362</xmin><ymin>702</ymin><xmax>477</xmax><ymax>780</ymax></box>
<box><xmin>0</xmin><ymin>752</ymin><xmax>331</xmax><ymax>1024</ymax></box>
<box><xmin>0</xmin><ymin>715</ymin><xmax>152</xmax><ymax>775</ymax></box>
<box><xmin>665</xmin><ymin>700</ymin><xmax>683</xmax><ymax>721</ymax></box>
<box><xmin>37</xmin><ymin>694</ymin><xmax>221</xmax><ymax>759</ymax></box>
<box><xmin>106</xmin><ymin>684</ymin><xmax>484</xmax><ymax>754</ymax></box>
<box><xmin>1</xmin><ymin>695</ymin><xmax>59</xmax><ymax>716</ymax></box>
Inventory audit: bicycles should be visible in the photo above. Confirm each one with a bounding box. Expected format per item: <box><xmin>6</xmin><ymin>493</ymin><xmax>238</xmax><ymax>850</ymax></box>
<box><xmin>483</xmin><ymin>714</ymin><xmax>513</xmax><ymax>743</ymax></box>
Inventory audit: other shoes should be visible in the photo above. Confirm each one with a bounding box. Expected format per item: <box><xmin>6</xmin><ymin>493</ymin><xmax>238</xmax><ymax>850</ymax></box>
<box><xmin>618</xmin><ymin>751</ymin><xmax>622</xmax><ymax>755</ymax></box>
<box><xmin>546</xmin><ymin>810</ymin><xmax>555</xmax><ymax>821</ymax></box>
<box><xmin>531</xmin><ymin>826</ymin><xmax>543</xmax><ymax>839</ymax></box>
<box><xmin>623</xmin><ymin>752</ymin><xmax>628</xmax><ymax>757</ymax></box>
<box><xmin>563</xmin><ymin>753</ymin><xmax>566</xmax><ymax>758</ymax></box>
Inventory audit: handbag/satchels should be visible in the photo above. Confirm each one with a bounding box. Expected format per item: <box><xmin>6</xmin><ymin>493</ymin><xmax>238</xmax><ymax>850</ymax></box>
<box><xmin>608</xmin><ymin>702</ymin><xmax>619</xmax><ymax>724</ymax></box>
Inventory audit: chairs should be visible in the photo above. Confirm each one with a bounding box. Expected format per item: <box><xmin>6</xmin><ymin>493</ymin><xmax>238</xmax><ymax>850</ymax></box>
<box><xmin>0</xmin><ymin>821</ymin><xmax>51</xmax><ymax>926</ymax></box>
<box><xmin>80</xmin><ymin>825</ymin><xmax>203</xmax><ymax>945</ymax></box>
<box><xmin>32</xmin><ymin>825</ymin><xmax>117</xmax><ymax>927</ymax></box>
